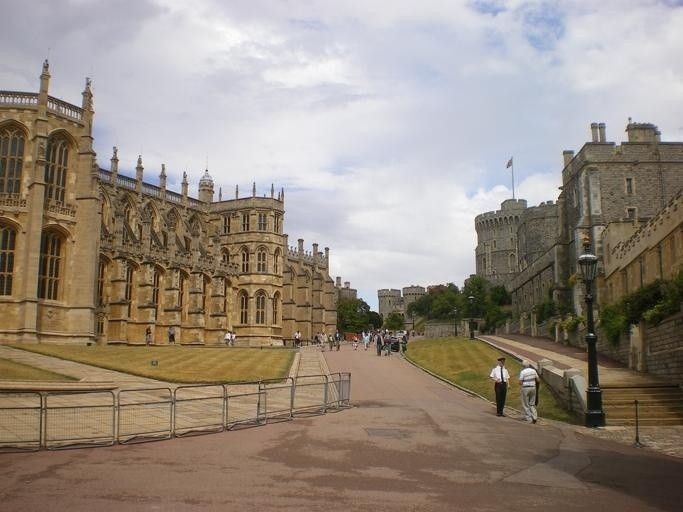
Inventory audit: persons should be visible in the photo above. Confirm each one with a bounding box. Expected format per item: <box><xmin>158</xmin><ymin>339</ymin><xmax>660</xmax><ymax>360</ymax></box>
<box><xmin>489</xmin><ymin>356</ymin><xmax>510</xmax><ymax>417</ymax></box>
<box><xmin>230</xmin><ymin>332</ymin><xmax>235</xmax><ymax>343</ymax></box>
<box><xmin>223</xmin><ymin>331</ymin><xmax>230</xmax><ymax>345</ymax></box>
<box><xmin>144</xmin><ymin>325</ymin><xmax>151</xmax><ymax>344</ymax></box>
<box><xmin>517</xmin><ymin>359</ymin><xmax>541</xmax><ymax>423</ymax></box>
<box><xmin>167</xmin><ymin>326</ymin><xmax>175</xmax><ymax>342</ymax></box>
<box><xmin>293</xmin><ymin>326</ymin><xmax>410</xmax><ymax>357</ymax></box>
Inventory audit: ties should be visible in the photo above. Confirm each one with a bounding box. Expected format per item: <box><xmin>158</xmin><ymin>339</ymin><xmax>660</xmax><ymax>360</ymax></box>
<box><xmin>501</xmin><ymin>367</ymin><xmax>504</xmax><ymax>383</ymax></box>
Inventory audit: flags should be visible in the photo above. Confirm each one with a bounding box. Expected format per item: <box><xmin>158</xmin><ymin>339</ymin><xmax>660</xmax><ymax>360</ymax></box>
<box><xmin>506</xmin><ymin>159</ymin><xmax>512</xmax><ymax>168</ymax></box>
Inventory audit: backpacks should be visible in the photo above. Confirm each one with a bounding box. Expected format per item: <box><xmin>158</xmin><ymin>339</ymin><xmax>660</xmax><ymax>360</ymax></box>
<box><xmin>377</xmin><ymin>335</ymin><xmax>381</xmax><ymax>345</ymax></box>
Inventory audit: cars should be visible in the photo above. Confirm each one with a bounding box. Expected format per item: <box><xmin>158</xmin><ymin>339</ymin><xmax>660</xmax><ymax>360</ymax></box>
<box><xmin>391</xmin><ymin>330</ymin><xmax>410</xmax><ymax>352</ymax></box>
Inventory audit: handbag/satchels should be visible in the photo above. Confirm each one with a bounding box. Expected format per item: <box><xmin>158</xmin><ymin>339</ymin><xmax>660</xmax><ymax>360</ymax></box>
<box><xmin>384</xmin><ymin>337</ymin><xmax>392</xmax><ymax>344</ymax></box>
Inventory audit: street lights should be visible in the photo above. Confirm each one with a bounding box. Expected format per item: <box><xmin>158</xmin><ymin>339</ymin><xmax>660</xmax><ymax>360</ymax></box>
<box><xmin>575</xmin><ymin>237</ymin><xmax>606</xmax><ymax>427</ymax></box>
<box><xmin>467</xmin><ymin>290</ymin><xmax>476</xmax><ymax>339</ymax></box>
<box><xmin>453</xmin><ymin>304</ymin><xmax>458</xmax><ymax>337</ymax></box>
<box><xmin>411</xmin><ymin>309</ymin><xmax>415</xmax><ymax>337</ymax></box>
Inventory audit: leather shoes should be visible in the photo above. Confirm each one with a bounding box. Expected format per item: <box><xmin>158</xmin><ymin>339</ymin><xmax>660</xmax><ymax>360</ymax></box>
<box><xmin>496</xmin><ymin>414</ymin><xmax>506</xmax><ymax>417</ymax></box>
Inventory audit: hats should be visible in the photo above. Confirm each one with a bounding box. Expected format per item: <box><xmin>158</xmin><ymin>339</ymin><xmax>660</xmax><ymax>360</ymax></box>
<box><xmin>497</xmin><ymin>358</ymin><xmax>505</xmax><ymax>361</ymax></box>
